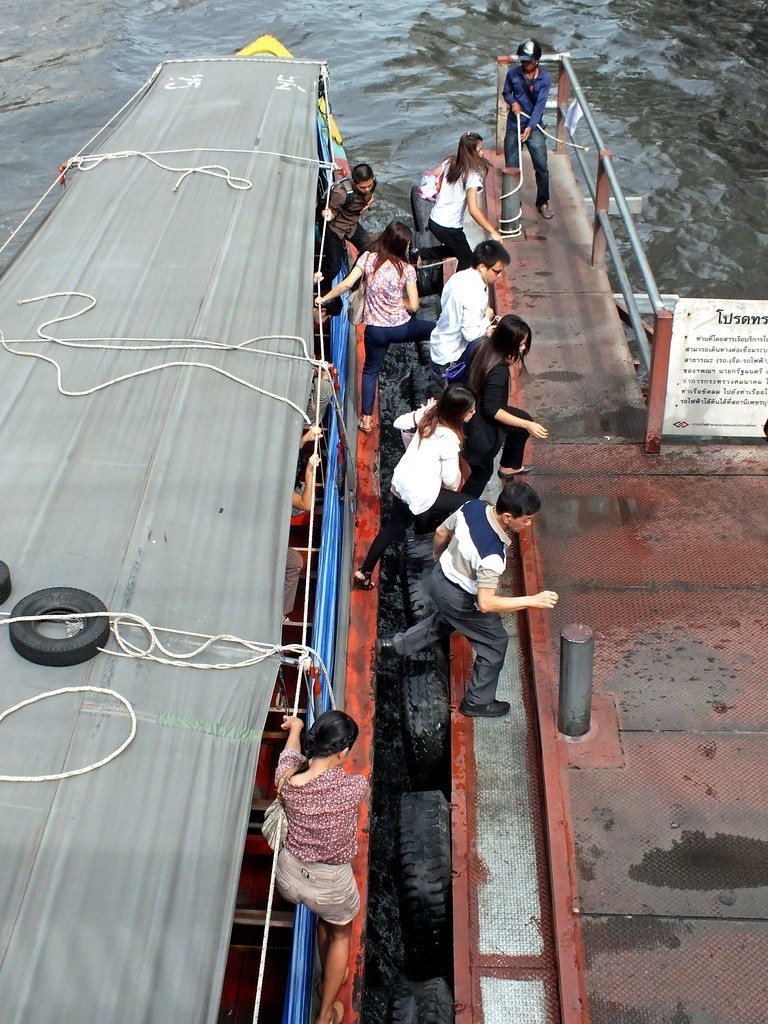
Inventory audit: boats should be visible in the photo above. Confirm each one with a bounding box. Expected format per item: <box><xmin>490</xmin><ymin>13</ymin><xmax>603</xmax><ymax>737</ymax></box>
<box><xmin>0</xmin><ymin>32</ymin><xmax>381</xmax><ymax>1024</ymax></box>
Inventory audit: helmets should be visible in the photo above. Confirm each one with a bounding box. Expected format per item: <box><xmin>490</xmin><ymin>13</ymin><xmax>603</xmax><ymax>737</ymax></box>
<box><xmin>516</xmin><ymin>37</ymin><xmax>542</xmax><ymax>62</ymax></box>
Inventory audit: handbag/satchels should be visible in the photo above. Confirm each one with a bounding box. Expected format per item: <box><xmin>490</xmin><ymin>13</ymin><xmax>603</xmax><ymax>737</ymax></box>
<box><xmin>261</xmin><ymin>767</ymin><xmax>297</xmax><ymax>854</ymax></box>
<box><xmin>419</xmin><ymin>157</ymin><xmax>452</xmax><ymax>202</ymax></box>
<box><xmin>346</xmin><ymin>252</ymin><xmax>374</xmax><ymax>326</ymax></box>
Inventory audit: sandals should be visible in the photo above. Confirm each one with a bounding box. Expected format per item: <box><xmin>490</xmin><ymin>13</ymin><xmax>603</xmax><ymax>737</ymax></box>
<box><xmin>314</xmin><ymin>967</ymin><xmax>350</xmax><ymax>999</ymax></box>
<box><xmin>354</xmin><ymin>568</ymin><xmax>376</xmax><ymax>591</ymax></box>
<box><xmin>313</xmin><ymin>1000</ymin><xmax>344</xmax><ymax>1024</ymax></box>
<box><xmin>358</xmin><ymin>418</ymin><xmax>372</xmax><ymax>434</ymax></box>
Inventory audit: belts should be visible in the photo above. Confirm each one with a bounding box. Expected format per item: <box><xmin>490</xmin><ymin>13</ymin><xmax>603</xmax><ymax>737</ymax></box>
<box><xmin>437</xmin><ymin>561</ymin><xmax>479</xmax><ymax>596</ymax></box>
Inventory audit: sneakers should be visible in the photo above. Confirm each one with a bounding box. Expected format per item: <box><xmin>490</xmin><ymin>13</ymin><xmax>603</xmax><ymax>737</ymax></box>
<box><xmin>458</xmin><ymin>696</ymin><xmax>511</xmax><ymax>718</ymax></box>
<box><xmin>374</xmin><ymin>638</ymin><xmax>397</xmax><ymax>668</ymax></box>
<box><xmin>535</xmin><ymin>203</ymin><xmax>554</xmax><ymax>220</ymax></box>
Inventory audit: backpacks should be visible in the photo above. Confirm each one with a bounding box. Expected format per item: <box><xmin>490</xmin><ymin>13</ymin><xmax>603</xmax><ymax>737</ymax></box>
<box><xmin>315</xmin><ymin>177</ymin><xmax>354</xmax><ymax>226</ymax></box>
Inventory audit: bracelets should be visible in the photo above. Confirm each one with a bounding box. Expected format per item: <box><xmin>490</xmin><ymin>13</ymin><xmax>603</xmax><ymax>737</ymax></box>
<box><xmin>321</xmin><ymin>297</ymin><xmax>329</xmax><ymax>304</ymax></box>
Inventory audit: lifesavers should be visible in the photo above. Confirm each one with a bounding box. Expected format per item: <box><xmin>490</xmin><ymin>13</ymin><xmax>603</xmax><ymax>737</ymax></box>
<box><xmin>6</xmin><ymin>585</ymin><xmax>112</xmax><ymax>669</ymax></box>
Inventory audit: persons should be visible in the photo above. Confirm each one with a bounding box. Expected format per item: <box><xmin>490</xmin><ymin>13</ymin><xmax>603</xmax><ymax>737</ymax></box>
<box><xmin>282</xmin><ymin>291</ymin><xmax>343</xmax><ymax>623</ymax></box>
<box><xmin>376</xmin><ymin>482</ymin><xmax>559</xmax><ymax>717</ymax></box>
<box><xmin>501</xmin><ymin>38</ymin><xmax>555</xmax><ymax>220</ymax></box>
<box><xmin>410</xmin><ymin>131</ymin><xmax>503</xmax><ymax>271</ymax></box>
<box><xmin>272</xmin><ymin>711</ymin><xmax>370</xmax><ymax>1024</ymax></box>
<box><xmin>315</xmin><ymin>164</ymin><xmax>377</xmax><ymax>295</ymax></box>
<box><xmin>427</xmin><ymin>240</ymin><xmax>512</xmax><ymax>399</ymax></box>
<box><xmin>354</xmin><ymin>386</ymin><xmax>476</xmax><ymax>590</ymax></box>
<box><xmin>314</xmin><ymin>221</ymin><xmax>436</xmax><ymax>434</ymax></box>
<box><xmin>458</xmin><ymin>313</ymin><xmax>550</xmax><ymax>499</ymax></box>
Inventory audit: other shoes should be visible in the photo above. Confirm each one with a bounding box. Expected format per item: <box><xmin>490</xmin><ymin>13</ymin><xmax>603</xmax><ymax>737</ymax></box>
<box><xmin>409</xmin><ymin>248</ymin><xmax>420</xmax><ymax>275</ymax></box>
<box><xmin>283</xmin><ymin>616</ymin><xmax>293</xmax><ymax>623</ymax></box>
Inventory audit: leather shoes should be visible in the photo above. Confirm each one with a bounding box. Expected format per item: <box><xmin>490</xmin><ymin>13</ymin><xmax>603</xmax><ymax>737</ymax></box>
<box><xmin>498</xmin><ymin>464</ymin><xmax>534</xmax><ymax>480</ymax></box>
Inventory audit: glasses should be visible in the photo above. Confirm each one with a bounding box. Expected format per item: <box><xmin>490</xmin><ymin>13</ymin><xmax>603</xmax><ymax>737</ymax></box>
<box><xmin>491</xmin><ymin>268</ymin><xmax>503</xmax><ymax>276</ymax></box>
